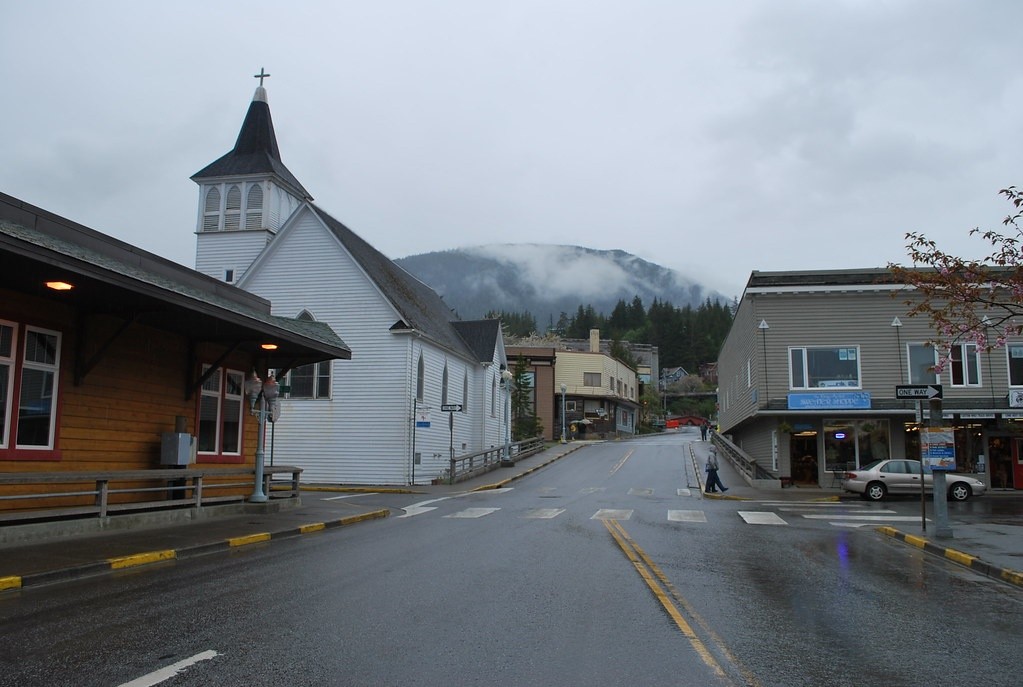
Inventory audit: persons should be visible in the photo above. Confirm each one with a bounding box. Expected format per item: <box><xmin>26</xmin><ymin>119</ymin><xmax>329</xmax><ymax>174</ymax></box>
<box><xmin>872</xmin><ymin>439</ymin><xmax>888</xmax><ymax>460</ymax></box>
<box><xmin>570</xmin><ymin>423</ymin><xmax>576</xmax><ymax>441</ymax></box>
<box><xmin>995</xmin><ymin>453</ymin><xmax>1011</xmax><ymax>489</ymax></box>
<box><xmin>700</xmin><ymin>421</ymin><xmax>708</xmax><ymax>441</ymax></box>
<box><xmin>705</xmin><ymin>444</ymin><xmax>729</xmax><ymax>494</ymax></box>
<box><xmin>826</xmin><ymin>446</ymin><xmax>840</xmax><ymax>463</ymax></box>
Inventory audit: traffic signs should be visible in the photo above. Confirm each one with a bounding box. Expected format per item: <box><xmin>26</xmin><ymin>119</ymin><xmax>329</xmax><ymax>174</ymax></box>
<box><xmin>896</xmin><ymin>384</ymin><xmax>944</xmax><ymax>401</ymax></box>
<box><xmin>441</xmin><ymin>404</ymin><xmax>462</xmax><ymax>412</ymax></box>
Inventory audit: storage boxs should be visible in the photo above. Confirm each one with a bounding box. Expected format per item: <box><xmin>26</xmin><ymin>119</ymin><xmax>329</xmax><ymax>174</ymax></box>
<box><xmin>818</xmin><ymin>380</ymin><xmax>858</xmax><ymax>387</ymax></box>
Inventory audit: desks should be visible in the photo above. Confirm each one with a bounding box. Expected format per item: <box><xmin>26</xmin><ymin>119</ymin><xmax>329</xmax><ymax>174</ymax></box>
<box><xmin>811</xmin><ymin>376</ymin><xmax>856</xmax><ymax>387</ymax></box>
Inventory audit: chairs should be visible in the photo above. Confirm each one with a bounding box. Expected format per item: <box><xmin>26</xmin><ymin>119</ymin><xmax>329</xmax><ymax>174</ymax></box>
<box><xmin>832</xmin><ymin>469</ymin><xmax>845</xmax><ymax>489</ymax></box>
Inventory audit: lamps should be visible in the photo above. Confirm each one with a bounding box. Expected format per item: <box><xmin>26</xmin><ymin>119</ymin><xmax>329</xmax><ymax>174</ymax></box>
<box><xmin>259</xmin><ymin>336</ymin><xmax>281</xmax><ymax>350</ymax></box>
<box><xmin>255</xmin><ymin>371</ymin><xmax>280</xmax><ymax>425</ymax></box>
<box><xmin>243</xmin><ymin>364</ymin><xmax>263</xmax><ymax>416</ymax></box>
<box><xmin>36</xmin><ymin>271</ymin><xmax>75</xmax><ymax>292</ymax></box>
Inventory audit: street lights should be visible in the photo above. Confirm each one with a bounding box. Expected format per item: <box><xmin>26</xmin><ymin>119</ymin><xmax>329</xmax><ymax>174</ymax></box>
<box><xmin>560</xmin><ymin>383</ymin><xmax>568</xmax><ymax>443</ymax></box>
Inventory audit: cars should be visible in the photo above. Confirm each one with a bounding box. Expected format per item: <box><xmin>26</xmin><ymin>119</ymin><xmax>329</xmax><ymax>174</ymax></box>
<box><xmin>840</xmin><ymin>458</ymin><xmax>987</xmax><ymax>502</ymax></box>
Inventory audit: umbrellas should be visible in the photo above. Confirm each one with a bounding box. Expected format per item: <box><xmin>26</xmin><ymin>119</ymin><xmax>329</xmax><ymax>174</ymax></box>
<box><xmin>569</xmin><ymin>418</ymin><xmax>594</xmax><ymax>424</ymax></box>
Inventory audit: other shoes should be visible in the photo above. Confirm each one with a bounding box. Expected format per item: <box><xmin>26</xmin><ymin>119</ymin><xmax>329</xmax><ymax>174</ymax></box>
<box><xmin>722</xmin><ymin>488</ymin><xmax>728</xmax><ymax>492</ymax></box>
<box><xmin>712</xmin><ymin>490</ymin><xmax>718</xmax><ymax>492</ymax></box>
<box><xmin>705</xmin><ymin>491</ymin><xmax>711</xmax><ymax>493</ymax></box>
